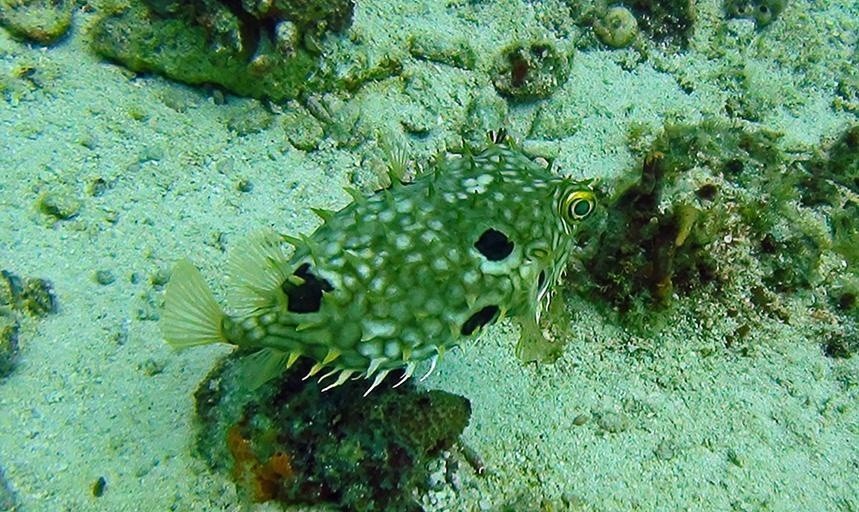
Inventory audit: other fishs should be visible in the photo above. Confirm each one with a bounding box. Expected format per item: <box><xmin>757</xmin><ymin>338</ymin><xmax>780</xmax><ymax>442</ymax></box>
<box><xmin>159</xmin><ymin>130</ymin><xmax>599</xmax><ymax>397</ymax></box>
